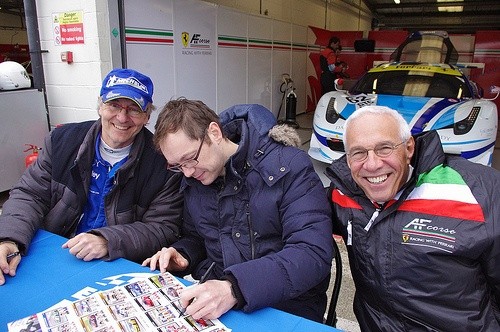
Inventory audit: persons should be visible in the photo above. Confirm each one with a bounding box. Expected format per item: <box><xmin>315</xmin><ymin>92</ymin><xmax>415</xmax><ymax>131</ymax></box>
<box><xmin>324</xmin><ymin>106</ymin><xmax>500</xmax><ymax>332</ymax></box>
<box><xmin>141</xmin><ymin>95</ymin><xmax>333</xmax><ymax>319</ymax></box>
<box><xmin>0</xmin><ymin>69</ymin><xmax>184</xmax><ymax>286</ymax></box>
<box><xmin>320</xmin><ymin>37</ymin><xmax>346</xmax><ymax>96</ymax></box>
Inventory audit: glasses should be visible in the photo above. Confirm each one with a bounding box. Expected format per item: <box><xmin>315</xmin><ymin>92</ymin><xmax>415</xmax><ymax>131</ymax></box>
<box><xmin>106</xmin><ymin>102</ymin><xmax>141</xmax><ymax>116</ymax></box>
<box><xmin>346</xmin><ymin>141</ymin><xmax>408</xmax><ymax>161</ymax></box>
<box><xmin>167</xmin><ymin>134</ymin><xmax>206</xmax><ymax>173</ymax></box>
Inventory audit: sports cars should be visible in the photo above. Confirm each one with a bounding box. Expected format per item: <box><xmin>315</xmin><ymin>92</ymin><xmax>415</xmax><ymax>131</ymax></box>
<box><xmin>307</xmin><ymin>29</ymin><xmax>498</xmax><ymax>187</ymax></box>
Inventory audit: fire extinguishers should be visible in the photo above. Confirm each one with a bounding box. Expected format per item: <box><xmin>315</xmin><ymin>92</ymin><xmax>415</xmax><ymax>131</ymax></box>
<box><xmin>23</xmin><ymin>143</ymin><xmax>42</xmax><ymax>168</ymax></box>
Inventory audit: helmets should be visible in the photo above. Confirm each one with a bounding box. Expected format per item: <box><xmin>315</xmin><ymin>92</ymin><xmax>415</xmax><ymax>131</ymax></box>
<box><xmin>0</xmin><ymin>60</ymin><xmax>32</xmax><ymax>89</ymax></box>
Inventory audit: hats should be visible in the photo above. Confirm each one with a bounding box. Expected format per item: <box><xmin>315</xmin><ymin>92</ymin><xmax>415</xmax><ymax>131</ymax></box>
<box><xmin>100</xmin><ymin>68</ymin><xmax>154</xmax><ymax>112</ymax></box>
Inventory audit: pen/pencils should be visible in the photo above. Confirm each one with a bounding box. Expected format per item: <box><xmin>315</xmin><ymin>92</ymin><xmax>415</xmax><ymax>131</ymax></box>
<box><xmin>4</xmin><ymin>252</ymin><xmax>20</xmax><ymax>261</ymax></box>
<box><xmin>178</xmin><ymin>261</ymin><xmax>217</xmax><ymax>319</ymax></box>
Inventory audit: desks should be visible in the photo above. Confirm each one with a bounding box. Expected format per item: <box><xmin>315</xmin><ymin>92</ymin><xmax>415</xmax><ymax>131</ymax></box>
<box><xmin>0</xmin><ymin>229</ymin><xmax>346</xmax><ymax>332</ymax></box>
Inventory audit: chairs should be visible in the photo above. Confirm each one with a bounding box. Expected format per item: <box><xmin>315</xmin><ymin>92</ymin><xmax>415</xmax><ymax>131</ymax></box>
<box><xmin>318</xmin><ymin>236</ymin><xmax>342</xmax><ymax>328</ymax></box>
